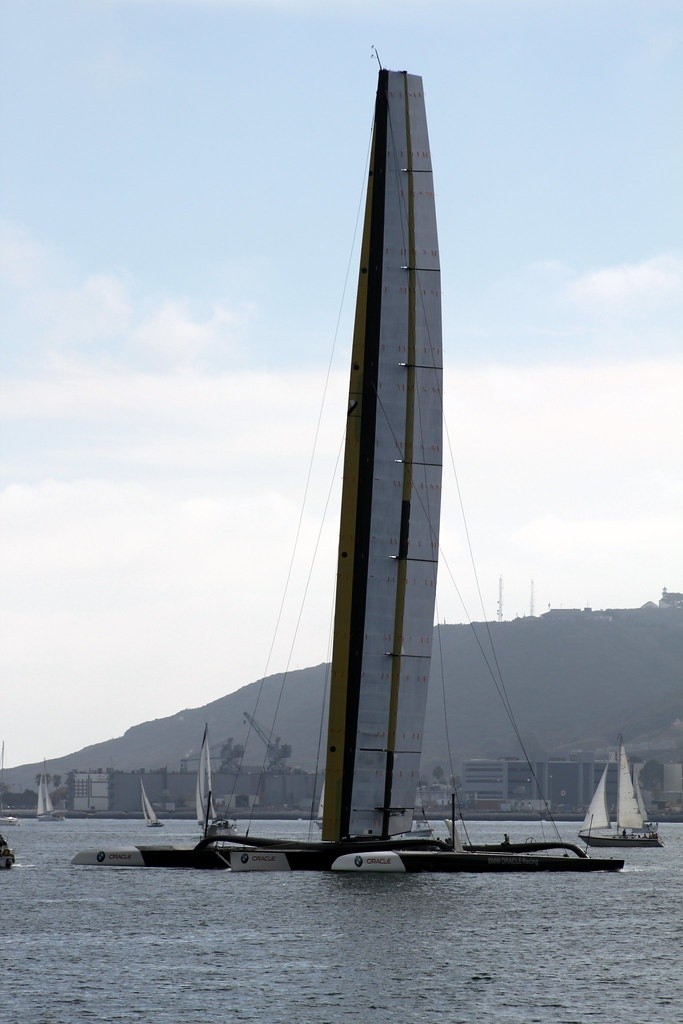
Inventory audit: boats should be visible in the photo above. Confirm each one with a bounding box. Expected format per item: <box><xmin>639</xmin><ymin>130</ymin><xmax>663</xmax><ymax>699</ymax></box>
<box><xmin>0</xmin><ymin>833</ymin><xmax>17</xmax><ymax>869</ymax></box>
<box><xmin>0</xmin><ymin>740</ymin><xmax>18</xmax><ymax>826</ymax></box>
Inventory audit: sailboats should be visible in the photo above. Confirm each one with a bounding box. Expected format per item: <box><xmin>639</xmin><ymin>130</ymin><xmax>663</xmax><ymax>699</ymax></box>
<box><xmin>139</xmin><ymin>776</ymin><xmax>165</xmax><ymax>827</ymax></box>
<box><xmin>70</xmin><ymin>62</ymin><xmax>629</xmax><ymax>878</ymax></box>
<box><xmin>194</xmin><ymin>721</ymin><xmax>238</xmax><ymax>836</ymax></box>
<box><xmin>35</xmin><ymin>756</ymin><xmax>63</xmax><ymax>821</ymax></box>
<box><xmin>578</xmin><ymin>732</ymin><xmax>664</xmax><ymax>848</ymax></box>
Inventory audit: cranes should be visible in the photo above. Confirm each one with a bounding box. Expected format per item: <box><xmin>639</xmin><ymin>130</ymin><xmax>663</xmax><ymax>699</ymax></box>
<box><xmin>242</xmin><ymin>709</ymin><xmax>293</xmax><ymax>777</ymax></box>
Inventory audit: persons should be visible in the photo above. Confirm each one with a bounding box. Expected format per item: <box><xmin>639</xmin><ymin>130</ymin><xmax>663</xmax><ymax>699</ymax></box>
<box><xmin>0</xmin><ymin>835</ymin><xmax>10</xmax><ymax>857</ymax></box>
<box><xmin>623</xmin><ymin>829</ymin><xmax>626</xmax><ymax>837</ymax></box>
